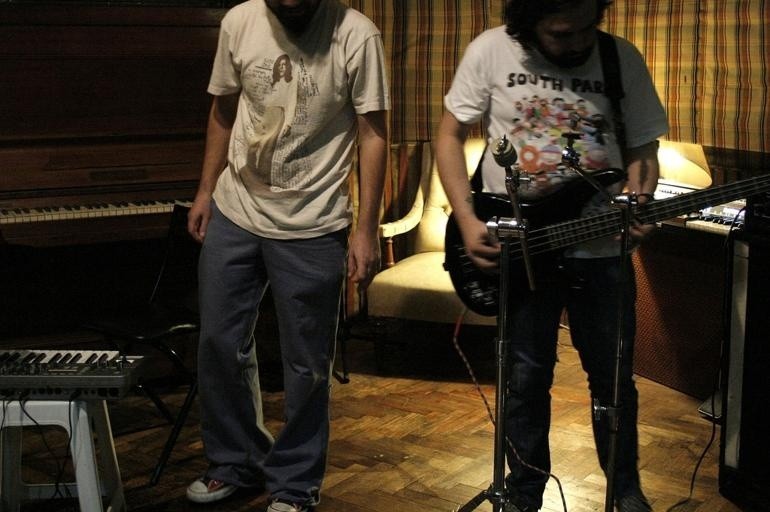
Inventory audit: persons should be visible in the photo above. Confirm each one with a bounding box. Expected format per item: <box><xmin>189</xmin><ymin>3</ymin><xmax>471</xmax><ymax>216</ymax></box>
<box><xmin>432</xmin><ymin>3</ymin><xmax>660</xmax><ymax>512</ymax></box>
<box><xmin>185</xmin><ymin>1</ymin><xmax>388</xmax><ymax>512</ymax></box>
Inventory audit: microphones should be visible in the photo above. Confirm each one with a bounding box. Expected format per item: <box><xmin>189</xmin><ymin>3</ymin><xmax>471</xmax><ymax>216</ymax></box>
<box><xmin>588</xmin><ymin>113</ymin><xmax>611</xmax><ymax>134</ymax></box>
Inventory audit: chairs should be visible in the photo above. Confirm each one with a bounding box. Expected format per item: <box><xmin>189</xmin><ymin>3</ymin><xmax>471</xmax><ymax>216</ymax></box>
<box><xmin>142</xmin><ymin>202</ymin><xmax>240</xmax><ymax>486</ymax></box>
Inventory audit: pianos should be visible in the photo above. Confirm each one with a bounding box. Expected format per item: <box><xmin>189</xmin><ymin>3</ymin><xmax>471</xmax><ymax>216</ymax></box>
<box><xmin>614</xmin><ymin>178</ymin><xmax>769</xmax><ymax>237</ymax></box>
<box><xmin>0</xmin><ymin>0</ymin><xmax>246</xmax><ymax>250</ymax></box>
<box><xmin>0</xmin><ymin>345</ymin><xmax>150</xmax><ymax>401</ymax></box>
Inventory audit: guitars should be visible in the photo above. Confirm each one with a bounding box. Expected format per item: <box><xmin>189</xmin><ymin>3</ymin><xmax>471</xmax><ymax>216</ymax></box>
<box><xmin>443</xmin><ymin>171</ymin><xmax>769</xmax><ymax>316</ymax></box>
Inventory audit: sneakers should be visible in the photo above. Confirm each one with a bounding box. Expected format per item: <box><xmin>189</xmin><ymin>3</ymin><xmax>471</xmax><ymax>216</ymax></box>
<box><xmin>266</xmin><ymin>498</ymin><xmax>308</xmax><ymax>512</ymax></box>
<box><xmin>186</xmin><ymin>476</ymin><xmax>239</xmax><ymax>503</ymax></box>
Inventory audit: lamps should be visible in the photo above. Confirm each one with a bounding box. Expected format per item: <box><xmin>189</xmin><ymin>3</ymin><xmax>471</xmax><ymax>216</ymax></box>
<box><xmin>657</xmin><ymin>139</ymin><xmax>713</xmax><ymax>191</ymax></box>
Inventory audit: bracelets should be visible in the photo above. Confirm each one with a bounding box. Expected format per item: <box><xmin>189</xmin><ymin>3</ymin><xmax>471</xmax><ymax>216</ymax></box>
<box><xmin>631</xmin><ymin>190</ymin><xmax>654</xmax><ymax>203</ymax></box>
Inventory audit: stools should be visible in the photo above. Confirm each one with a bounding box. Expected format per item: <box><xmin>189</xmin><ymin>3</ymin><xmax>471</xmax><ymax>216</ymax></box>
<box><xmin>0</xmin><ymin>398</ymin><xmax>130</xmax><ymax>511</ymax></box>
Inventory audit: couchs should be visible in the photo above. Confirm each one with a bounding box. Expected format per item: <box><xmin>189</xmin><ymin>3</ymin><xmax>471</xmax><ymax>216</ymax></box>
<box><xmin>365</xmin><ymin>142</ymin><xmax>513</xmax><ymax>372</ymax></box>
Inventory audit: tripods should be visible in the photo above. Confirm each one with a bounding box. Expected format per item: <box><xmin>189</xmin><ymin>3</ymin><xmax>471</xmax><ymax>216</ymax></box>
<box><xmin>457</xmin><ymin>237</ymin><xmax>533</xmax><ymax>512</ymax></box>
